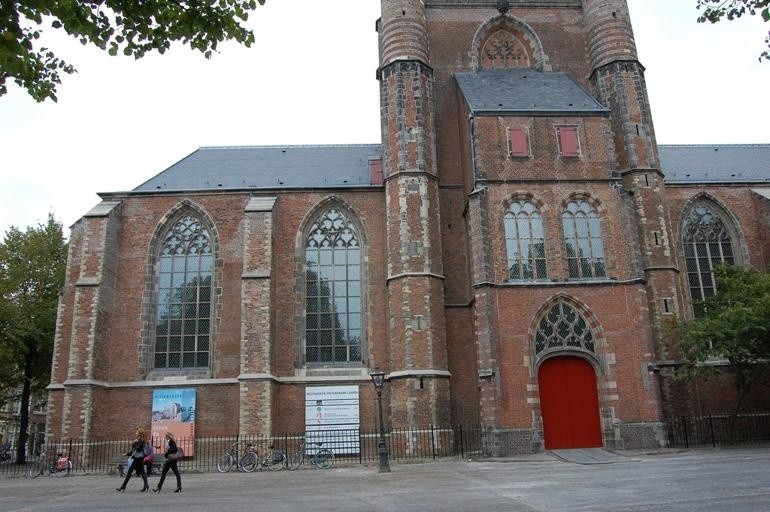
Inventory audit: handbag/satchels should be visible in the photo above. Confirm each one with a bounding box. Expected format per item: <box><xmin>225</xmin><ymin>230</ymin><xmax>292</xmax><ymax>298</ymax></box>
<box><xmin>132</xmin><ymin>448</ymin><xmax>144</xmax><ymax>459</ymax></box>
<box><xmin>167</xmin><ymin>447</ymin><xmax>185</xmax><ymax>462</ymax></box>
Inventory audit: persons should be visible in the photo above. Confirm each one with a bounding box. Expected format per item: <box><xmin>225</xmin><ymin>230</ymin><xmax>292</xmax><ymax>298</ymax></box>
<box><xmin>135</xmin><ymin>442</ymin><xmax>153</xmax><ymax>477</ymax></box>
<box><xmin>116</xmin><ymin>427</ymin><xmax>149</xmax><ymax>491</ymax></box>
<box><xmin>0</xmin><ymin>439</ymin><xmax>12</xmax><ymax>462</ymax></box>
<box><xmin>152</xmin><ymin>432</ymin><xmax>182</xmax><ymax>492</ymax></box>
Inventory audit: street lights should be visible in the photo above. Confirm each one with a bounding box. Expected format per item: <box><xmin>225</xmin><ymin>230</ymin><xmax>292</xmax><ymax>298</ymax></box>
<box><xmin>369</xmin><ymin>365</ymin><xmax>392</xmax><ymax>474</ymax></box>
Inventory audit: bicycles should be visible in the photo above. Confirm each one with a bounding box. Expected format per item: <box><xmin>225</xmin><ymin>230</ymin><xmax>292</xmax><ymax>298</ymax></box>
<box><xmin>286</xmin><ymin>441</ymin><xmax>336</xmax><ymax>471</ymax></box>
<box><xmin>216</xmin><ymin>442</ymin><xmax>287</xmax><ymax>473</ymax></box>
<box><xmin>29</xmin><ymin>449</ymin><xmax>73</xmax><ymax>479</ymax></box>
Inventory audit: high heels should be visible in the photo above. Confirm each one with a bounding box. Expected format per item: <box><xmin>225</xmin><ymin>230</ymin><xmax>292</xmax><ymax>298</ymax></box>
<box><xmin>116</xmin><ymin>486</ymin><xmax>125</xmax><ymax>492</ymax></box>
<box><xmin>140</xmin><ymin>486</ymin><xmax>149</xmax><ymax>492</ymax></box>
<box><xmin>174</xmin><ymin>486</ymin><xmax>182</xmax><ymax>493</ymax></box>
<box><xmin>153</xmin><ymin>487</ymin><xmax>161</xmax><ymax>492</ymax></box>
